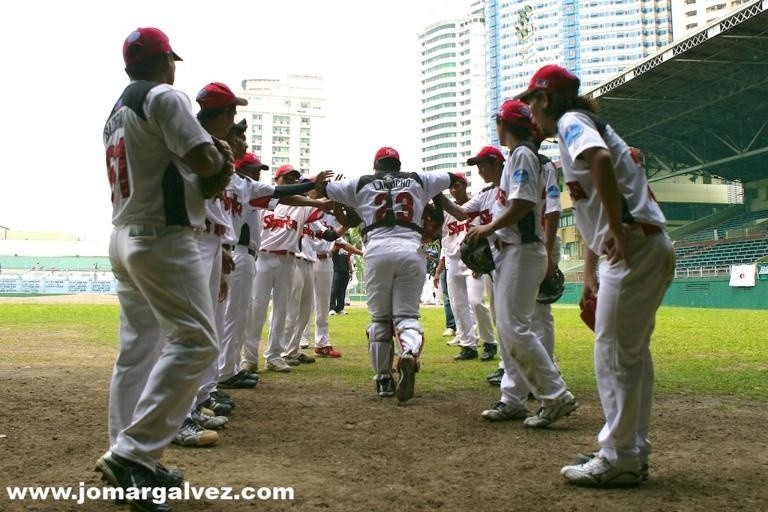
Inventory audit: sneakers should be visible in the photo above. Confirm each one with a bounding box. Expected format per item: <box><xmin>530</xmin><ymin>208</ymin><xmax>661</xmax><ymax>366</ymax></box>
<box><xmin>396</xmin><ymin>354</ymin><xmax>417</xmax><ymax>402</ymax></box>
<box><xmin>94</xmin><ymin>310</ymin><xmax>348</xmax><ymax>511</ymax></box>
<box><xmin>442</xmin><ymin>328</ymin><xmax>649</xmax><ymax>486</ymax></box>
<box><xmin>376</xmin><ymin>378</ymin><xmax>397</xmax><ymax>397</ymax></box>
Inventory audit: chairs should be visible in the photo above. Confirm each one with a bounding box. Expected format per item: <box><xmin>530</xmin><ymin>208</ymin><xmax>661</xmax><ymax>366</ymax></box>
<box><xmin>675</xmin><ymin>208</ymin><xmax>768</xmax><ymax>277</ymax></box>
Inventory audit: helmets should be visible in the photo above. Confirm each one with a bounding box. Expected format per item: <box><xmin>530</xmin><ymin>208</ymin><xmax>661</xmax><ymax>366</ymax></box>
<box><xmin>459</xmin><ymin>234</ymin><xmax>495</xmax><ymax>275</ymax></box>
<box><xmin>536</xmin><ymin>263</ymin><xmax>564</xmax><ymax>303</ymax></box>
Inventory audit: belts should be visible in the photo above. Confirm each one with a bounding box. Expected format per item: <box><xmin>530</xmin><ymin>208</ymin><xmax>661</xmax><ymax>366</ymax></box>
<box><xmin>316</xmin><ymin>252</ymin><xmax>332</xmax><ymax>259</ymax></box>
<box><xmin>606</xmin><ymin>224</ymin><xmax>661</xmax><ymax>249</ymax></box>
<box><xmin>232</xmin><ymin>245</ymin><xmax>258</xmax><ymax>260</ymax></box>
<box><xmin>296</xmin><ymin>256</ymin><xmax>314</xmax><ymax>263</ymax></box>
<box><xmin>202</xmin><ymin>222</ymin><xmax>226</xmax><ymax>236</ymax></box>
<box><xmin>263</xmin><ymin>248</ymin><xmax>295</xmax><ymax>256</ymax></box>
<box><xmin>495</xmin><ymin>235</ymin><xmax>542</xmax><ymax>251</ymax></box>
<box><xmin>222</xmin><ymin>243</ymin><xmax>235</xmax><ymax>252</ymax></box>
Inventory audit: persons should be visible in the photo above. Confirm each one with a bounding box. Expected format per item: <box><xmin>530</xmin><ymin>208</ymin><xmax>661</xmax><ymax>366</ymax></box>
<box><xmin>171</xmin><ymin>83</ymin><xmax>579</xmax><ymax>446</ymax></box>
<box><xmin>512</xmin><ymin>65</ymin><xmax>677</xmax><ymax>488</ymax></box>
<box><xmin>95</xmin><ymin>28</ymin><xmax>235</xmax><ymax>511</ymax></box>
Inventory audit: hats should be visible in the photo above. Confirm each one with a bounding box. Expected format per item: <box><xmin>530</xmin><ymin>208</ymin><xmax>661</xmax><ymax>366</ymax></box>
<box><xmin>512</xmin><ymin>64</ymin><xmax>580</xmax><ymax>104</ymax></box>
<box><xmin>196</xmin><ymin>83</ymin><xmax>248</xmax><ymax>111</ymax></box>
<box><xmin>467</xmin><ymin>147</ymin><xmax>505</xmax><ymax>165</ymax></box>
<box><xmin>298</xmin><ymin>174</ymin><xmax>311</xmax><ymax>183</ymax></box>
<box><xmin>375</xmin><ymin>146</ymin><xmax>401</xmax><ymax>164</ymax></box>
<box><xmin>491</xmin><ymin>99</ymin><xmax>531</xmax><ymax>128</ymax></box>
<box><xmin>218</xmin><ymin>122</ymin><xmax>247</xmax><ymax>139</ymax></box>
<box><xmin>275</xmin><ymin>165</ymin><xmax>301</xmax><ymax>179</ymax></box>
<box><xmin>449</xmin><ymin>172</ymin><xmax>467</xmax><ymax>185</ymax></box>
<box><xmin>236</xmin><ymin>152</ymin><xmax>269</xmax><ymax>171</ymax></box>
<box><xmin>123</xmin><ymin>27</ymin><xmax>184</xmax><ymax>64</ymax></box>
